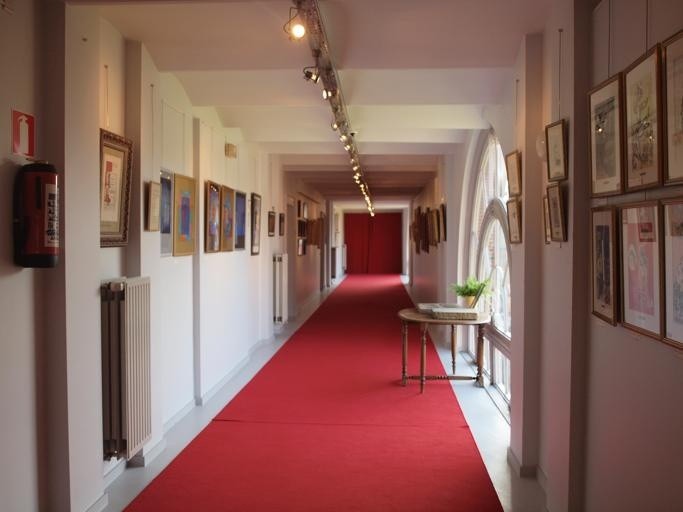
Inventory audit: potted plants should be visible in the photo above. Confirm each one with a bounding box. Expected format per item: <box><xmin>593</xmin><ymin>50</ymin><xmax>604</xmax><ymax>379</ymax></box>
<box><xmin>448</xmin><ymin>274</ymin><xmax>493</xmax><ymax>308</ymax></box>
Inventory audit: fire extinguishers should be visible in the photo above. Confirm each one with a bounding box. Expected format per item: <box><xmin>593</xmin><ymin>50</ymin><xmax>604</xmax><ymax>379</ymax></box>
<box><xmin>13</xmin><ymin>157</ymin><xmax>60</xmax><ymax>268</ymax></box>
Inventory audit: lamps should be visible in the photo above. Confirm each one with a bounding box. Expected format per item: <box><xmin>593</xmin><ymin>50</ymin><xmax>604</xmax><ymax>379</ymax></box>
<box><xmin>283</xmin><ymin>0</ymin><xmax>377</xmax><ymax>218</ymax></box>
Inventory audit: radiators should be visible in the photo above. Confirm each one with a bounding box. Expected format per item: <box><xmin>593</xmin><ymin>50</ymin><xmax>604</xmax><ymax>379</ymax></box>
<box><xmin>342</xmin><ymin>244</ymin><xmax>348</xmax><ymax>270</ymax></box>
<box><xmin>100</xmin><ymin>275</ymin><xmax>153</xmax><ymax>461</ymax></box>
<box><xmin>273</xmin><ymin>252</ymin><xmax>289</xmax><ymax>325</ymax></box>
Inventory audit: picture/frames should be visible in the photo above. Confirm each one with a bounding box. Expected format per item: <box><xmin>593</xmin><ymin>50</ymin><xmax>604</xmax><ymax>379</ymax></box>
<box><xmin>414</xmin><ymin>204</ymin><xmax>446</xmax><ymax>254</ymax></box>
<box><xmin>586</xmin><ymin>28</ymin><xmax>682</xmax><ymax>349</ymax></box>
<box><xmin>506</xmin><ymin>199</ymin><xmax>522</xmax><ymax>244</ymax></box>
<box><xmin>280</xmin><ymin>213</ymin><xmax>285</xmax><ymax>236</ymax></box>
<box><xmin>542</xmin><ymin>118</ymin><xmax>568</xmax><ymax>244</ymax></box>
<box><xmin>145</xmin><ymin>173</ymin><xmax>262</xmax><ymax>257</ymax></box>
<box><xmin>99</xmin><ymin>126</ymin><xmax>134</xmax><ymax>248</ymax></box>
<box><xmin>268</xmin><ymin>210</ymin><xmax>275</xmax><ymax>237</ymax></box>
<box><xmin>504</xmin><ymin>150</ymin><xmax>522</xmax><ymax>196</ymax></box>
